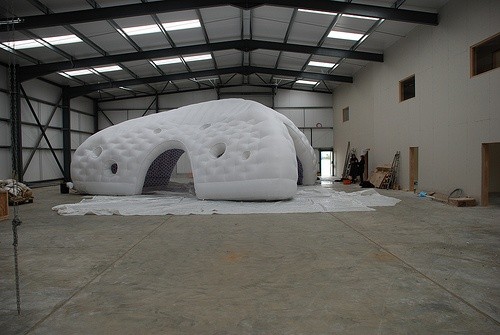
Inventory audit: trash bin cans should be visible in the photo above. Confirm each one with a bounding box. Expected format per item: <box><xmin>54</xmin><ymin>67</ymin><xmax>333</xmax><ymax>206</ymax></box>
<box><xmin>60</xmin><ymin>182</ymin><xmax>69</xmax><ymax>194</ymax></box>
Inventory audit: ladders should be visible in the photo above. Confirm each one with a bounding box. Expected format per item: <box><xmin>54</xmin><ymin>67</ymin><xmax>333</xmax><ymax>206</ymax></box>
<box><xmin>379</xmin><ymin>150</ymin><xmax>400</xmax><ymax>189</ymax></box>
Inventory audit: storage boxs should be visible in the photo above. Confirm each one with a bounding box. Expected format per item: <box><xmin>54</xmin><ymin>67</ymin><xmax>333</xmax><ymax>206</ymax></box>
<box><xmin>343</xmin><ymin>180</ymin><xmax>350</xmax><ymax>185</ymax></box>
<box><xmin>0</xmin><ymin>191</ymin><xmax>9</xmax><ymax>221</ymax></box>
<box><xmin>449</xmin><ymin>198</ymin><xmax>475</xmax><ymax>207</ymax></box>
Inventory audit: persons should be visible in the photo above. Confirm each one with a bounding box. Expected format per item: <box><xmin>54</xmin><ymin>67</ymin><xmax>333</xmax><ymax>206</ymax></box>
<box><xmin>350</xmin><ymin>154</ymin><xmax>358</xmax><ymax>184</ymax></box>
<box><xmin>357</xmin><ymin>155</ymin><xmax>365</xmax><ymax>186</ymax></box>
